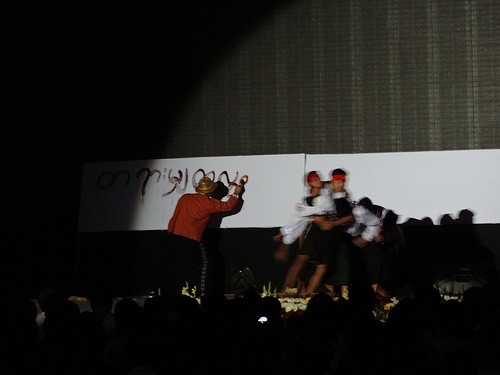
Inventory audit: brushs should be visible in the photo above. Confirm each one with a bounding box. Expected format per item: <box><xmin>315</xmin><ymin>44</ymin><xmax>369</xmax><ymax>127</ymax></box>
<box><xmin>239</xmin><ymin>179</ymin><xmax>244</xmax><ymax>186</ymax></box>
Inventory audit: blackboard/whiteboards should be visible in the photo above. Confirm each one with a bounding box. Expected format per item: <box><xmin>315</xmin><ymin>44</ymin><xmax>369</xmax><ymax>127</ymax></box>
<box><xmin>79</xmin><ymin>149</ymin><xmax>500</xmax><ymax>231</ymax></box>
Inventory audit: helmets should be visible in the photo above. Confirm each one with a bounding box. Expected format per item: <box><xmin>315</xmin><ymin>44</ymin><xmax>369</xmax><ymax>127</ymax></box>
<box><xmin>195</xmin><ymin>177</ymin><xmax>218</xmax><ymax>194</ymax></box>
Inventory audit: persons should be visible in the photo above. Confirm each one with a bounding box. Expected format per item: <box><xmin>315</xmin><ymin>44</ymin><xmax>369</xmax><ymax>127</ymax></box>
<box><xmin>272</xmin><ymin>169</ymin><xmax>405</xmax><ymax>301</ymax></box>
<box><xmin>152</xmin><ymin>176</ymin><xmax>245</xmax><ymax>303</ymax></box>
<box><xmin>0</xmin><ymin>285</ymin><xmax>500</xmax><ymax>375</ymax></box>
